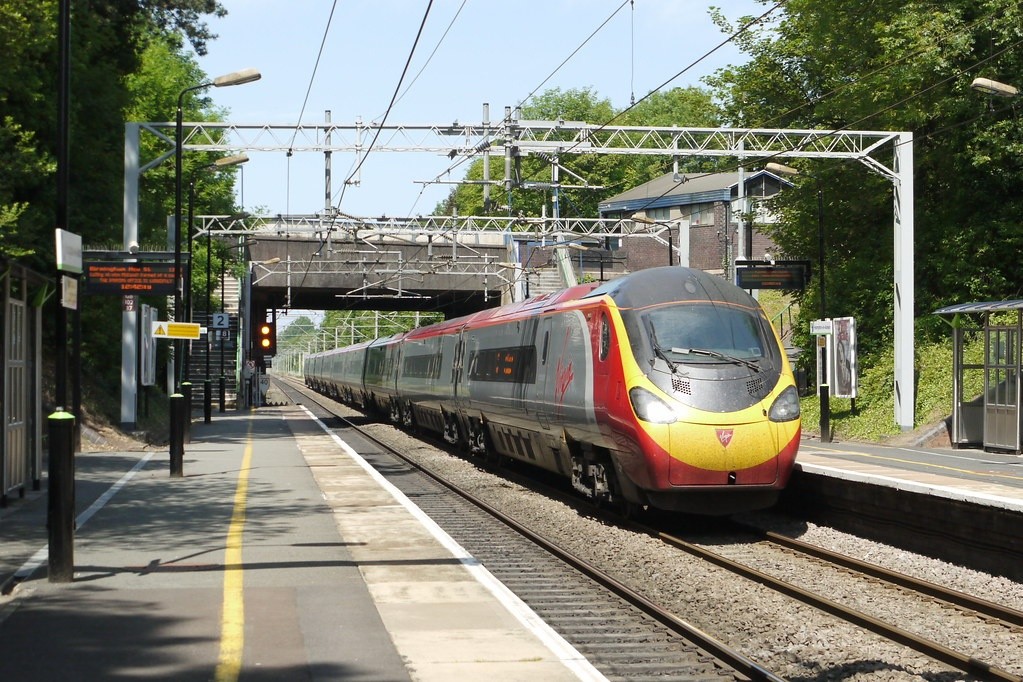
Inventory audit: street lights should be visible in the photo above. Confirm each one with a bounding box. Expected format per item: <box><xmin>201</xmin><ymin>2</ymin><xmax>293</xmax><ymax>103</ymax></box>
<box><xmin>630</xmin><ymin>214</ymin><xmax>674</xmax><ymax>266</ymax></box>
<box><xmin>218</xmin><ymin>240</ymin><xmax>258</xmax><ymax>415</ymax></box>
<box><xmin>203</xmin><ymin>211</ymin><xmax>252</xmax><ymax>424</ymax></box>
<box><xmin>499</xmin><ymin>262</ymin><xmax>530</xmax><ymax>298</ymax></box>
<box><xmin>766</xmin><ymin>160</ymin><xmax>832</xmax><ymax>441</ymax></box>
<box><xmin>186</xmin><ymin>153</ymin><xmax>250</xmax><ymax>429</ymax></box>
<box><xmin>161</xmin><ymin>66</ymin><xmax>262</xmax><ymax>480</ymax></box>
<box><xmin>569</xmin><ymin>242</ymin><xmax>604</xmax><ymax>282</ymax></box>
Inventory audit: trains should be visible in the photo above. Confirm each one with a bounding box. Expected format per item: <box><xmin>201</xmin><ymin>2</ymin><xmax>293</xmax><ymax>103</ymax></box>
<box><xmin>301</xmin><ymin>264</ymin><xmax>802</xmax><ymax>515</ymax></box>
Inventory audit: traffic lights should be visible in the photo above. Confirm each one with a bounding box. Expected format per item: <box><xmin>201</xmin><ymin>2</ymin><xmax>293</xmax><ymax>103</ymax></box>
<box><xmin>255</xmin><ymin>322</ymin><xmax>276</xmax><ymax>357</ymax></box>
